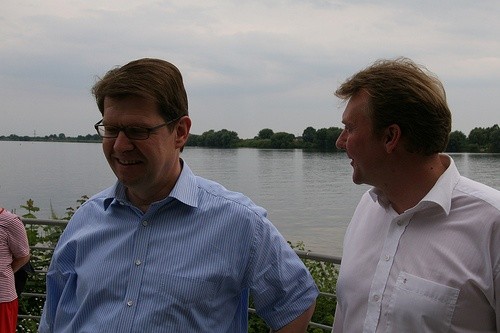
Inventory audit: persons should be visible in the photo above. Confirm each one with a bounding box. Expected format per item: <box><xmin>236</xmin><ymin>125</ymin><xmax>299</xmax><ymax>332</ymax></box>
<box><xmin>0</xmin><ymin>206</ymin><xmax>30</xmax><ymax>333</ymax></box>
<box><xmin>36</xmin><ymin>58</ymin><xmax>320</xmax><ymax>333</ymax></box>
<box><xmin>331</xmin><ymin>57</ymin><xmax>500</xmax><ymax>333</ymax></box>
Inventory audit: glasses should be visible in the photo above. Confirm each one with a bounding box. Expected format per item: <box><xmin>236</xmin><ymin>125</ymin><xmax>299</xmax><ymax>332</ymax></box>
<box><xmin>94</xmin><ymin>115</ymin><xmax>183</xmax><ymax>140</ymax></box>
